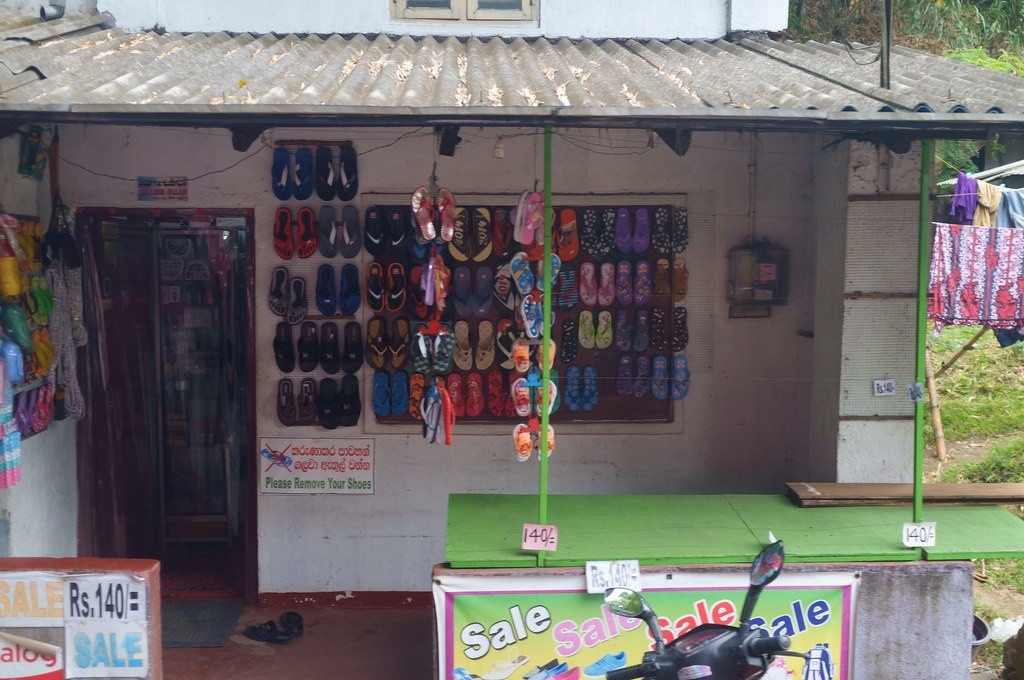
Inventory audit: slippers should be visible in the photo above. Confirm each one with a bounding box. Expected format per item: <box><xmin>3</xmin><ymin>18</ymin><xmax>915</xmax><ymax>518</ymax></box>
<box><xmin>293</xmin><ymin>148</ymin><xmax>313</xmax><ymax>200</ymax></box>
<box><xmin>28</xmin><ymin>273</ymin><xmax>53</xmax><ymax>326</ymax></box>
<box><xmin>337</xmin><ymin>146</ymin><xmax>358</xmax><ymax>201</ymax></box>
<box><xmin>2</xmin><ymin>304</ymin><xmax>32</xmax><ymax>348</ymax></box>
<box><xmin>31</xmin><ymin>327</ymin><xmax>56</xmax><ymax>379</ymax></box>
<box><xmin>14</xmin><ymin>389</ymin><xmax>37</xmax><ymax>435</ymax></box>
<box><xmin>268</xmin><ymin>185</ymin><xmax>701</xmax><ymax>463</ymax></box>
<box><xmin>272</xmin><ymin>147</ymin><xmax>292</xmax><ymax>200</ymax></box>
<box><xmin>314</xmin><ymin>146</ymin><xmax>337</xmax><ymax>201</ymax></box>
<box><xmin>31</xmin><ymin>383</ymin><xmax>54</xmax><ymax>432</ymax></box>
<box><xmin>0</xmin><ymin>339</ymin><xmax>24</xmax><ymax>384</ymax></box>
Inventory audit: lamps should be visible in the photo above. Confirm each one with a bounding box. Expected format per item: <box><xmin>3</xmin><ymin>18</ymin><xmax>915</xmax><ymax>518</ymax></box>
<box><xmin>492</xmin><ymin>138</ymin><xmax>504</xmax><ymax>160</ymax></box>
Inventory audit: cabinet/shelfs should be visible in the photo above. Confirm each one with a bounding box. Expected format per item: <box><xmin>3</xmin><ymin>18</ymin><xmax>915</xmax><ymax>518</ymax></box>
<box><xmin>150</xmin><ymin>229</ymin><xmax>236</xmax><ymax>552</ymax></box>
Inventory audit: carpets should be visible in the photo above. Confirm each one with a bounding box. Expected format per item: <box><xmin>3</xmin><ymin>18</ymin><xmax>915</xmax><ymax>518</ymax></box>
<box><xmin>161</xmin><ymin>594</ymin><xmax>250</xmax><ymax>650</ymax></box>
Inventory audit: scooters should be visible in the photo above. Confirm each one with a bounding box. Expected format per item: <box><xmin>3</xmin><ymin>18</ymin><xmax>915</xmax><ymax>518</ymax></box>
<box><xmin>602</xmin><ymin>540</ymin><xmax>791</xmax><ymax>680</ymax></box>
